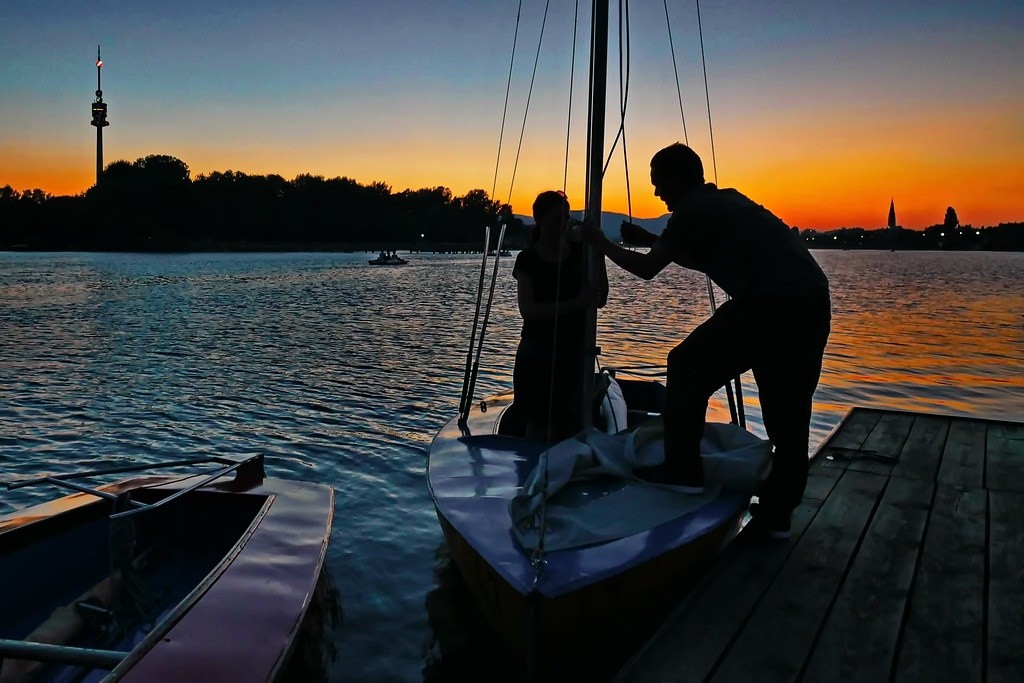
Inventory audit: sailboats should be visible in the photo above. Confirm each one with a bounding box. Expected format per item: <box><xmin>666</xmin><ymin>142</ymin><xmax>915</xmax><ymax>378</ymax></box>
<box><xmin>421</xmin><ymin>0</ymin><xmax>777</xmax><ymax>673</ymax></box>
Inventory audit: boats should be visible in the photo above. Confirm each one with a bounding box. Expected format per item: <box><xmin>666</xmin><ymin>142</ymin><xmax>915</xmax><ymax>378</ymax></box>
<box><xmin>369</xmin><ymin>253</ymin><xmax>409</xmax><ymax>267</ymax></box>
<box><xmin>0</xmin><ymin>447</ymin><xmax>334</xmax><ymax>683</ymax></box>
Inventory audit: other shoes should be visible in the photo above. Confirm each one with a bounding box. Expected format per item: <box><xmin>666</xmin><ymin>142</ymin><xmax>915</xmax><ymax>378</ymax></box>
<box><xmin>631</xmin><ymin>458</ymin><xmax>705</xmax><ymax>493</ymax></box>
<box><xmin>749</xmin><ymin>503</ymin><xmax>792</xmax><ymax>539</ymax></box>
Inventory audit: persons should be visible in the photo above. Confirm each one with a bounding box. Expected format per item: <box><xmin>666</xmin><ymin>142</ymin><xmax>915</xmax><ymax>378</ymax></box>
<box><xmin>573</xmin><ymin>141</ymin><xmax>831</xmax><ymax>537</ymax></box>
<box><xmin>379</xmin><ymin>249</ymin><xmax>398</xmax><ymax>258</ymax></box>
<box><xmin>512</xmin><ymin>190</ymin><xmax>609</xmax><ymax>439</ymax></box>
